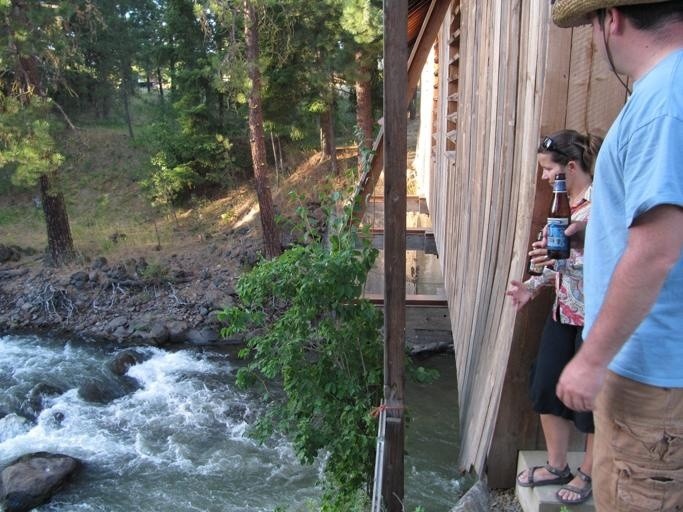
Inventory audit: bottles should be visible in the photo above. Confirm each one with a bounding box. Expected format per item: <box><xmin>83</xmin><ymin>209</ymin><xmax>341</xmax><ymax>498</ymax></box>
<box><xmin>545</xmin><ymin>172</ymin><xmax>572</xmax><ymax>260</ymax></box>
<box><xmin>527</xmin><ymin>230</ymin><xmax>546</xmax><ymax>276</ymax></box>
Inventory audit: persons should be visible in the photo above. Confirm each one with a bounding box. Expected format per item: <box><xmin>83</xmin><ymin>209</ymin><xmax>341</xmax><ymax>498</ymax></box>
<box><xmin>538</xmin><ymin>0</ymin><xmax>683</xmax><ymax>511</ymax></box>
<box><xmin>504</xmin><ymin>128</ymin><xmax>603</xmax><ymax>504</ymax></box>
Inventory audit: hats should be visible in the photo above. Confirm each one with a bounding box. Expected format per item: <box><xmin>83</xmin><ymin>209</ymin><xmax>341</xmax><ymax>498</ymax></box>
<box><xmin>552</xmin><ymin>0</ymin><xmax>660</xmax><ymax>26</ymax></box>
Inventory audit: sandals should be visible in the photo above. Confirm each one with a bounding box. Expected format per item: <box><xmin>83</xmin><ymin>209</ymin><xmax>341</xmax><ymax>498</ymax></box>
<box><xmin>517</xmin><ymin>462</ymin><xmax>574</xmax><ymax>486</ymax></box>
<box><xmin>557</xmin><ymin>467</ymin><xmax>593</xmax><ymax>504</ymax></box>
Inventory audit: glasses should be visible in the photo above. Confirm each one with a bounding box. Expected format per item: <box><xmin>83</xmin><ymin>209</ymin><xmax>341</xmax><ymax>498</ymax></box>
<box><xmin>543</xmin><ymin>135</ymin><xmax>568</xmax><ymax>158</ymax></box>
